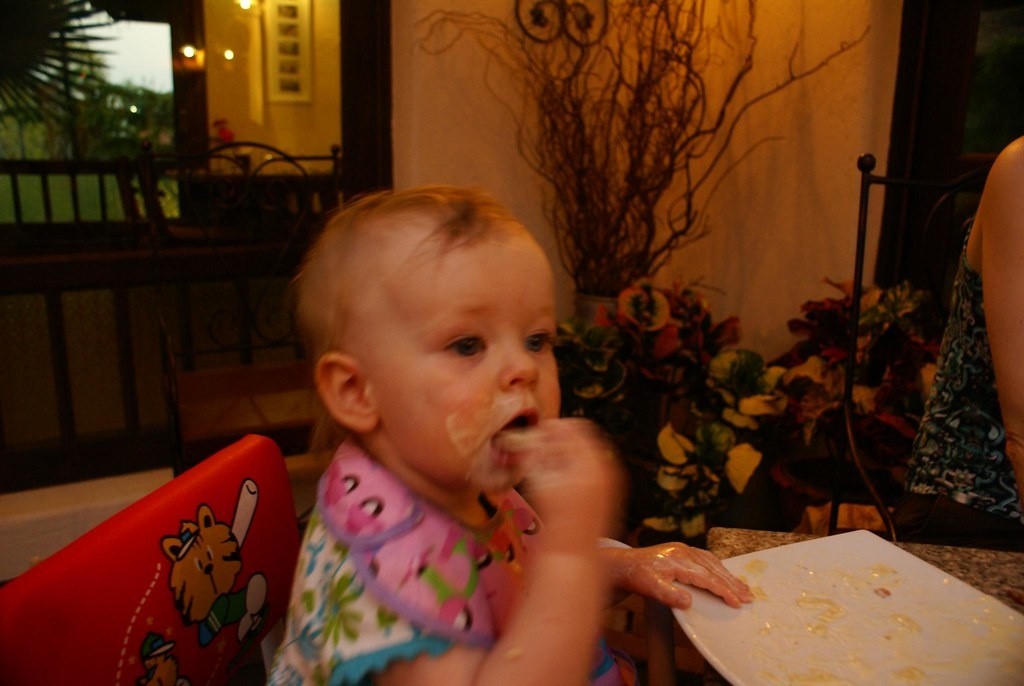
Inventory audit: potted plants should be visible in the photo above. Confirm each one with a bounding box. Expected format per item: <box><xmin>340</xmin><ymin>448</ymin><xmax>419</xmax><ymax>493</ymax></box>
<box><xmin>411</xmin><ymin>0</ymin><xmax>873</xmax><ymax>327</ymax></box>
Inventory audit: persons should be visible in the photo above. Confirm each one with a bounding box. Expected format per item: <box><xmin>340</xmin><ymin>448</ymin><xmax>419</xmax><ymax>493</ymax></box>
<box><xmin>896</xmin><ymin>133</ymin><xmax>1024</xmax><ymax>548</ymax></box>
<box><xmin>266</xmin><ymin>184</ymin><xmax>755</xmax><ymax>685</ymax></box>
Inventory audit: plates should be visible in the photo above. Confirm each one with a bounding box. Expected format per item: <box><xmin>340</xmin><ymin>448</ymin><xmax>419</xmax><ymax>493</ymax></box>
<box><xmin>667</xmin><ymin>529</ymin><xmax>1024</xmax><ymax>686</ymax></box>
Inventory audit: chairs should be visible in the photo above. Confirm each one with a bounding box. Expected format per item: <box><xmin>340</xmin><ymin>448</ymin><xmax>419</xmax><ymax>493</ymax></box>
<box><xmin>138</xmin><ymin>136</ymin><xmax>343</xmax><ymax>476</ymax></box>
<box><xmin>832</xmin><ymin>154</ymin><xmax>996</xmax><ymax>545</ymax></box>
<box><xmin>0</xmin><ymin>433</ymin><xmax>301</xmax><ymax>686</ymax></box>
